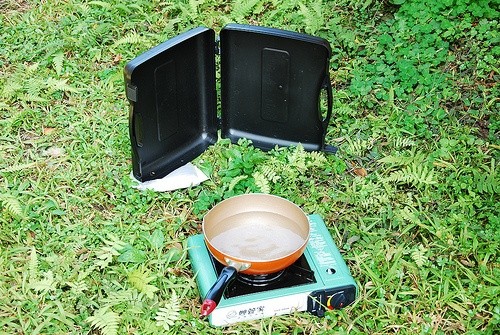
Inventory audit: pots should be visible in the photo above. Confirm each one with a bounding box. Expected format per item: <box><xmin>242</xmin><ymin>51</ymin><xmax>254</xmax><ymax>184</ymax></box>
<box><xmin>200</xmin><ymin>193</ymin><xmax>311</xmax><ymax>315</ymax></box>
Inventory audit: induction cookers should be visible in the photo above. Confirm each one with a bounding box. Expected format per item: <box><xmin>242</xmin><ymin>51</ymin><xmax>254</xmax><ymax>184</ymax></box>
<box><xmin>186</xmin><ymin>214</ymin><xmax>358</xmax><ymax>328</ymax></box>
